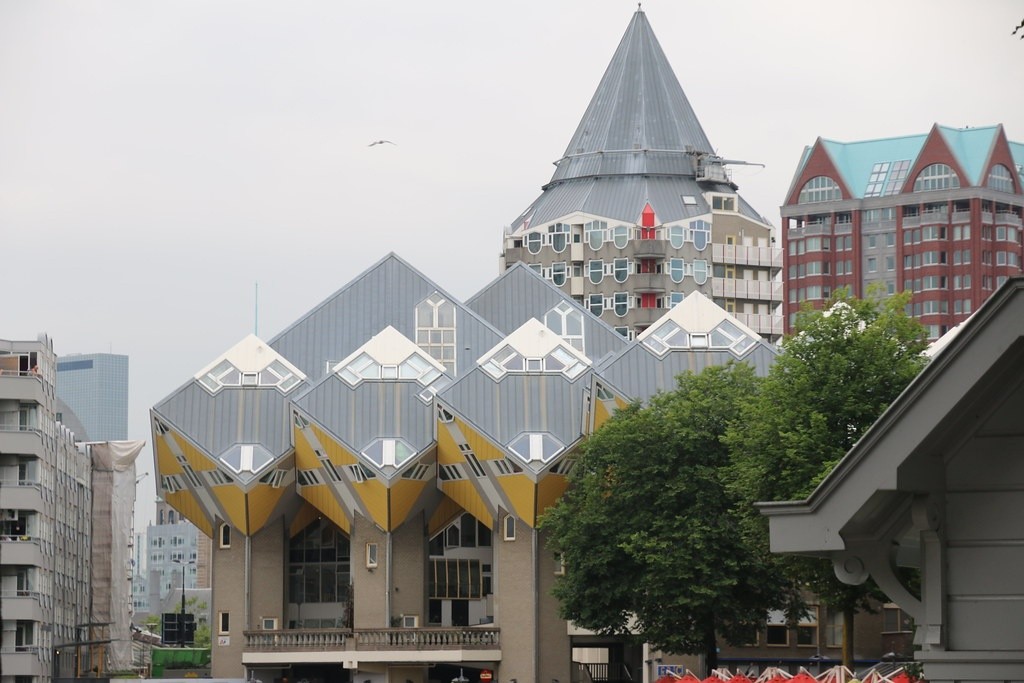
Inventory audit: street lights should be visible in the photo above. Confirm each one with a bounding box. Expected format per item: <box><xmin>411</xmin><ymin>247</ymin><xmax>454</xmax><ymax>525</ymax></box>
<box><xmin>173</xmin><ymin>559</ymin><xmax>197</xmax><ymax>648</ymax></box>
<box><xmin>147</xmin><ymin>622</ymin><xmax>158</xmax><ymax>680</ymax></box>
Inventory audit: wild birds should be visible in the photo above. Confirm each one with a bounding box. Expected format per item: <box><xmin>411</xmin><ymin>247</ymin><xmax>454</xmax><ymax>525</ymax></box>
<box><xmin>369</xmin><ymin>141</ymin><xmax>397</xmax><ymax>146</ymax></box>
